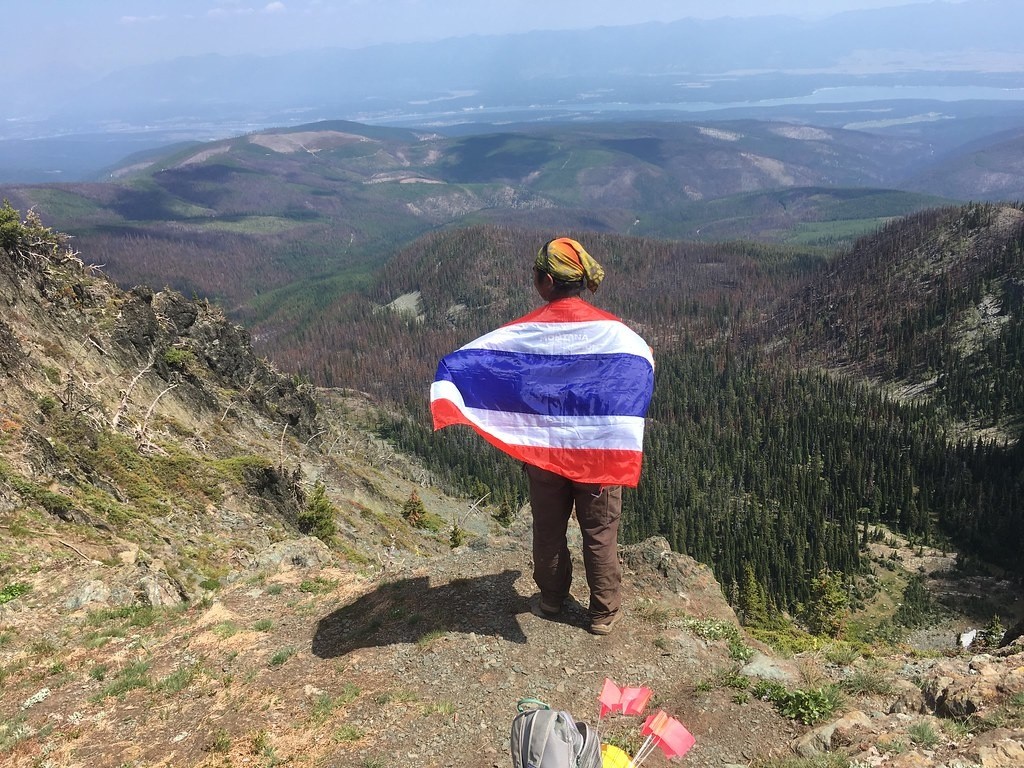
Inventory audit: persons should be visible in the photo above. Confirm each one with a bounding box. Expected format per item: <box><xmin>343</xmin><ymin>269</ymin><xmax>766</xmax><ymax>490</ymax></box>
<box><xmin>525</xmin><ymin>237</ymin><xmax>624</xmax><ymax>637</ymax></box>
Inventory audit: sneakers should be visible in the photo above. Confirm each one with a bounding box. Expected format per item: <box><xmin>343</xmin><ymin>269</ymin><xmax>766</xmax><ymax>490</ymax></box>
<box><xmin>539</xmin><ymin>597</ymin><xmax>561</xmax><ymax>614</ymax></box>
<box><xmin>590</xmin><ymin>608</ymin><xmax>623</xmax><ymax>635</ymax></box>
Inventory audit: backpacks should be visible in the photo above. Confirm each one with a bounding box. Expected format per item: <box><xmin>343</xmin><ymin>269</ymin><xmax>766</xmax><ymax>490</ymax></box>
<box><xmin>510</xmin><ymin>699</ymin><xmax>604</xmax><ymax>768</ymax></box>
